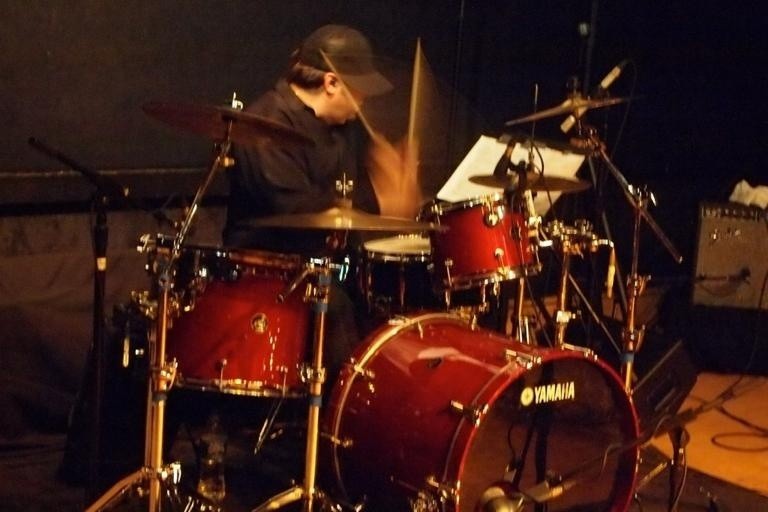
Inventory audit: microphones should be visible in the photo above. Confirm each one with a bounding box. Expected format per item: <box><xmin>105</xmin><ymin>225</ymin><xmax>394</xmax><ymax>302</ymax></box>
<box><xmin>740</xmin><ymin>268</ymin><xmax>751</xmax><ymax>285</ymax></box>
<box><xmin>559</xmin><ymin>60</ymin><xmax>629</xmax><ymax>135</ymax></box>
<box><xmin>180</xmin><ymin>192</ymin><xmax>190</xmax><ymax>221</ymax></box>
<box><xmin>493</xmin><ymin>135</ymin><xmax>520</xmax><ymax>177</ymax></box>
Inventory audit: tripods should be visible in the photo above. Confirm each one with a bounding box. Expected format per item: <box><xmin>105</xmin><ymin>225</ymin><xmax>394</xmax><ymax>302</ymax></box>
<box><xmin>629</xmin><ymin>425</ymin><xmax>723</xmax><ymax>512</ymax></box>
<box><xmin>249</xmin><ymin>242</ymin><xmax>344</xmax><ymax>512</ymax></box>
<box><xmin>82</xmin><ymin>147</ymin><xmax>226</xmax><ymax>512</ymax></box>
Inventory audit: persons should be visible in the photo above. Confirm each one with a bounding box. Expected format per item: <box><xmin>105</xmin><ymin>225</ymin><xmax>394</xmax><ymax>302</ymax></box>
<box><xmin>217</xmin><ymin>22</ymin><xmax>394</xmax><ymax>257</ymax></box>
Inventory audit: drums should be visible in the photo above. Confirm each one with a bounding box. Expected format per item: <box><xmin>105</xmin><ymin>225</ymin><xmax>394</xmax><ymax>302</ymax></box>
<box><xmin>360</xmin><ymin>236</ymin><xmax>432</xmax><ymax>309</ymax></box>
<box><xmin>166</xmin><ymin>246</ymin><xmax>315</xmax><ymax>398</ymax></box>
<box><xmin>318</xmin><ymin>312</ymin><xmax>642</xmax><ymax>511</ymax></box>
<box><xmin>430</xmin><ymin>194</ymin><xmax>541</xmax><ymax>291</ymax></box>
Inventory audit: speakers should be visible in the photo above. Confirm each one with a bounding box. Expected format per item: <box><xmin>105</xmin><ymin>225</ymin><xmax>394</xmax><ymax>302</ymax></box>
<box><xmin>535</xmin><ymin>306</ymin><xmax>697</xmax><ymax>446</ymax></box>
<box><xmin>690</xmin><ymin>201</ymin><xmax>768</xmax><ymax>316</ymax></box>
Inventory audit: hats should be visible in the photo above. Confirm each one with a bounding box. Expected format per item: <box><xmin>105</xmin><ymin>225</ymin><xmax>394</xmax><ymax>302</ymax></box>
<box><xmin>297</xmin><ymin>26</ymin><xmax>395</xmax><ymax>97</ymax></box>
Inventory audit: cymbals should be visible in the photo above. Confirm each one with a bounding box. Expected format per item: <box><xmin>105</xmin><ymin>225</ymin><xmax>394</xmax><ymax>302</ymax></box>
<box><xmin>239</xmin><ymin>207</ymin><xmax>444</xmax><ymax>235</ymax></box>
<box><xmin>469</xmin><ymin>175</ymin><xmax>591</xmax><ymax>191</ymax></box>
<box><xmin>142</xmin><ymin>100</ymin><xmax>313</xmax><ymax>145</ymax></box>
<box><xmin>506</xmin><ymin>99</ymin><xmax>625</xmax><ymax>126</ymax></box>
